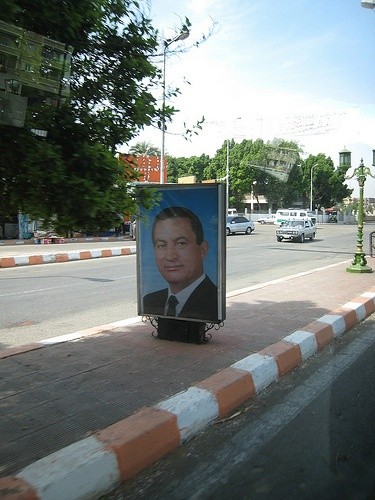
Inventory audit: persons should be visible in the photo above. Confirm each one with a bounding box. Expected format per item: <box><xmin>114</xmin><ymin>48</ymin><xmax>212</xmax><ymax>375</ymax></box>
<box><xmin>143</xmin><ymin>206</ymin><xmax>218</xmax><ymax>321</ymax></box>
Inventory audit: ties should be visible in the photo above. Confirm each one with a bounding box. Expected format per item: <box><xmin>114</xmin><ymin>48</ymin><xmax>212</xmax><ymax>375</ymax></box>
<box><xmin>167</xmin><ymin>295</ymin><xmax>179</xmax><ymax>317</ymax></box>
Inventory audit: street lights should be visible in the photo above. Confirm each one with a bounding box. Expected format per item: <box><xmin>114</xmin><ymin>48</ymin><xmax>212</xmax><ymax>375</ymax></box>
<box><xmin>311</xmin><ymin>164</ymin><xmax>318</xmax><ymax>211</ymax></box>
<box><xmin>225</xmin><ymin>116</ymin><xmax>242</xmax><ymax>217</ymax></box>
<box><xmin>160</xmin><ymin>32</ymin><xmax>190</xmax><ymax>183</ymax></box>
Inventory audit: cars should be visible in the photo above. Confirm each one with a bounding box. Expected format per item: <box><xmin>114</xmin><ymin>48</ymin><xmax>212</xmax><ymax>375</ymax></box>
<box><xmin>258</xmin><ymin>214</ymin><xmax>275</xmax><ymax>224</ymax></box>
<box><xmin>225</xmin><ymin>215</ymin><xmax>255</xmax><ymax>236</ymax></box>
<box><xmin>276</xmin><ymin>219</ymin><xmax>316</xmax><ymax>243</ymax></box>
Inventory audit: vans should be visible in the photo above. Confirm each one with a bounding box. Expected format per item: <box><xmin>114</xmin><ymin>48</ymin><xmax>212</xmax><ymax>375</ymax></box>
<box><xmin>274</xmin><ymin>209</ymin><xmax>316</xmax><ymax>226</ymax></box>
